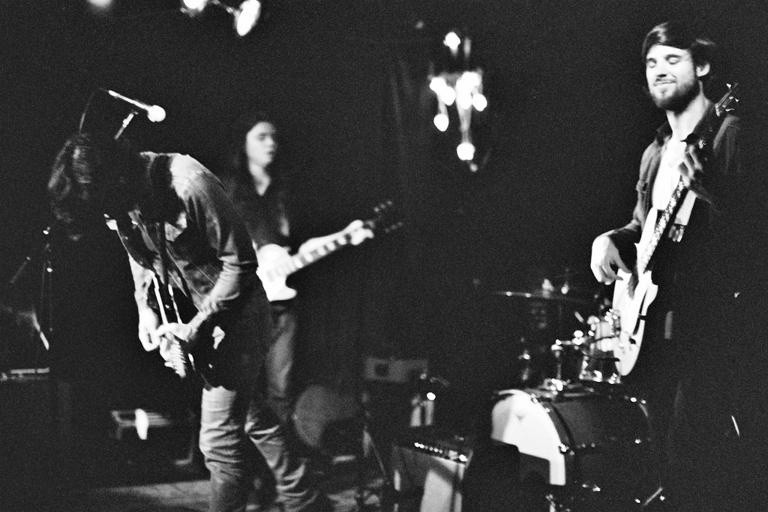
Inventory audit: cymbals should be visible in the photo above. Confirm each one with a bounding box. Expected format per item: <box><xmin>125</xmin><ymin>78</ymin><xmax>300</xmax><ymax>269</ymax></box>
<box><xmin>496</xmin><ymin>291</ymin><xmax>592</xmax><ymax>306</ymax></box>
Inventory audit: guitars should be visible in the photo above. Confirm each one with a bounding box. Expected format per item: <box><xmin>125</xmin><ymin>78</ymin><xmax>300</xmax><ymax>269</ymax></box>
<box><xmin>612</xmin><ymin>83</ymin><xmax>741</xmax><ymax>376</ymax></box>
<box><xmin>151</xmin><ymin>279</ymin><xmax>190</xmax><ymax>378</ymax></box>
<box><xmin>257</xmin><ymin>201</ymin><xmax>404</xmax><ymax>303</ymax></box>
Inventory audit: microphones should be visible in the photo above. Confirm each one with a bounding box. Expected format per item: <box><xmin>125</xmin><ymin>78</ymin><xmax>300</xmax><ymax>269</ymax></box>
<box><xmin>107</xmin><ymin>89</ymin><xmax>167</xmax><ymax>123</ymax></box>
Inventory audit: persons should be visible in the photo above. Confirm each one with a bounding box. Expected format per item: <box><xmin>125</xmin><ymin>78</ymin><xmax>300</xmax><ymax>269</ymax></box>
<box><xmin>588</xmin><ymin>23</ymin><xmax>767</xmax><ymax>512</ymax></box>
<box><xmin>47</xmin><ymin>132</ymin><xmax>337</xmax><ymax>511</ymax></box>
<box><xmin>223</xmin><ymin>114</ymin><xmax>375</xmax><ymax>490</ymax></box>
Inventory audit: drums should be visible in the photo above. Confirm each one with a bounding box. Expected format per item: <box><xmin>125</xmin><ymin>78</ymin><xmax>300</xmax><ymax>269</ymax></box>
<box><xmin>491</xmin><ymin>379</ymin><xmax>654</xmax><ymax>508</ymax></box>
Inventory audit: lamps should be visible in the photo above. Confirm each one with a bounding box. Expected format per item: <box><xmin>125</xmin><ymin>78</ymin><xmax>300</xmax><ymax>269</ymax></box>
<box><xmin>177</xmin><ymin>0</ymin><xmax>261</xmax><ymax>40</ymax></box>
